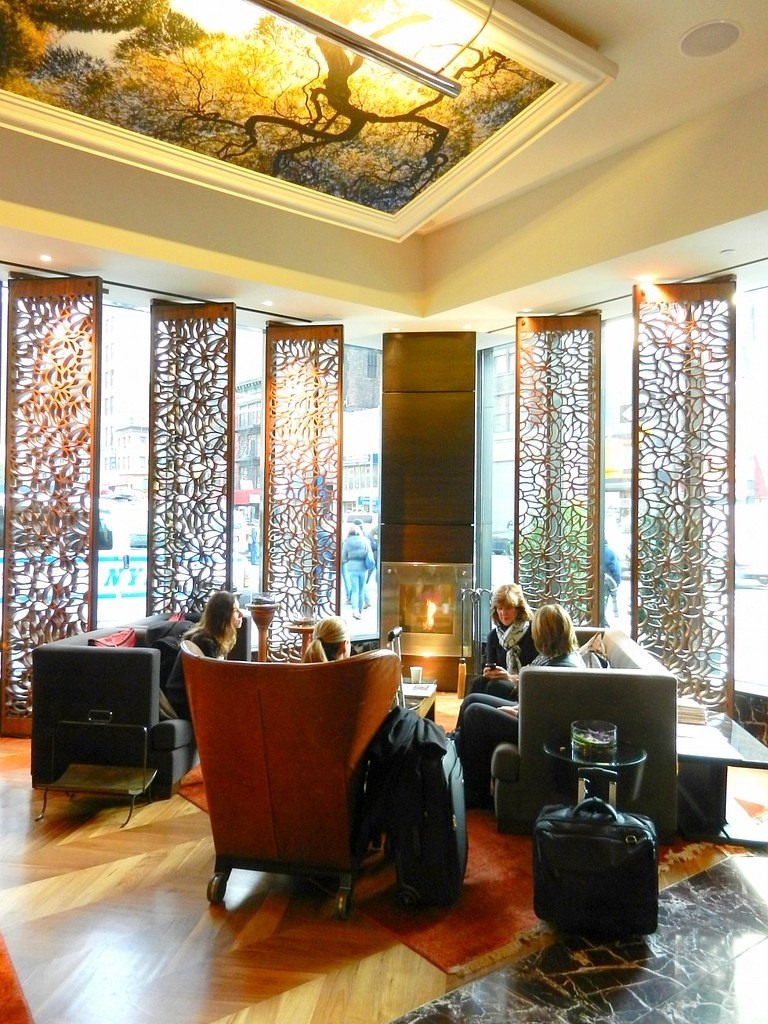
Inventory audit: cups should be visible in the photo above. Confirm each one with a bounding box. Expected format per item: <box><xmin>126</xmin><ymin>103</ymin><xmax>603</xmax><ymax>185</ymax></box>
<box><xmin>410</xmin><ymin>666</ymin><xmax>423</xmax><ymax>684</ymax></box>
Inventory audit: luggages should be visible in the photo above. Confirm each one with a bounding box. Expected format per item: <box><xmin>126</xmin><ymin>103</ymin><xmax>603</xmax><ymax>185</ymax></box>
<box><xmin>533</xmin><ymin>767</ymin><xmax>659</xmax><ymax>934</ymax></box>
<box><xmin>364</xmin><ymin>706</ymin><xmax>468</xmax><ymax>912</ymax></box>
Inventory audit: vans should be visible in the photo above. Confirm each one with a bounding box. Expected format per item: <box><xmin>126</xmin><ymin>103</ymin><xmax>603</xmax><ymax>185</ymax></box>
<box><xmin>202</xmin><ymin>508</ymin><xmax>249</xmax><ymax>552</ymax></box>
<box><xmin>343</xmin><ymin>513</ymin><xmax>380</xmax><ymax>537</ymax></box>
<box><xmin>0</xmin><ymin>489</ymin><xmax>254</xmax><ymax>648</ymax></box>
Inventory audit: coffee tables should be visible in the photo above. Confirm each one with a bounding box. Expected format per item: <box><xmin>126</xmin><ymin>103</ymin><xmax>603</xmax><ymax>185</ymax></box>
<box><xmin>392</xmin><ymin>678</ymin><xmax>437</xmax><ymax>728</ymax></box>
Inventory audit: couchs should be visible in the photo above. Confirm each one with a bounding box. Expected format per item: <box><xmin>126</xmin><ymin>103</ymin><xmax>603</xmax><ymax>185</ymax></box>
<box><xmin>29</xmin><ymin>611</ymin><xmax>255</xmax><ymax>798</ymax></box>
<box><xmin>484</xmin><ymin>621</ymin><xmax>679</xmax><ymax>848</ymax></box>
<box><xmin>178</xmin><ymin>640</ymin><xmax>407</xmax><ymax>926</ymax></box>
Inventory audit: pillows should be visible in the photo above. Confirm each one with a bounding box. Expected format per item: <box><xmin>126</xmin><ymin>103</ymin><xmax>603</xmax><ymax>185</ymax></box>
<box><xmin>169</xmin><ymin>609</ymin><xmax>188</xmax><ymax>623</ymax></box>
<box><xmin>581</xmin><ymin>650</ymin><xmax>614</xmax><ymax>667</ymax></box>
<box><xmin>579</xmin><ymin>629</ymin><xmax>605</xmax><ymax>655</ymax></box>
<box><xmin>86</xmin><ymin>625</ymin><xmax>136</xmax><ymax>648</ymax></box>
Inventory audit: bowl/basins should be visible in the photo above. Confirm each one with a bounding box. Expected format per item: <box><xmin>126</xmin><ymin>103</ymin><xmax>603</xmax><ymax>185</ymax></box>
<box><xmin>570</xmin><ymin>720</ymin><xmax>618</xmax><ymax>748</ymax></box>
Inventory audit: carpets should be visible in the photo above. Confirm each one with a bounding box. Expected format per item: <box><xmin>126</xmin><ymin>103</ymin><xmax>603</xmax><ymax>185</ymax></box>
<box><xmin>0</xmin><ymin>926</ymin><xmax>37</xmax><ymax>1024</ymax></box>
<box><xmin>175</xmin><ymin>704</ymin><xmax>727</xmax><ymax>979</ymax></box>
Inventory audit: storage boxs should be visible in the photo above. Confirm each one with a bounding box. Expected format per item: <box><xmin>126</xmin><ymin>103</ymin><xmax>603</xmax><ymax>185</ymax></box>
<box><xmin>380</xmin><ymin>561</ymin><xmax>472</xmax><ymax>657</ymax></box>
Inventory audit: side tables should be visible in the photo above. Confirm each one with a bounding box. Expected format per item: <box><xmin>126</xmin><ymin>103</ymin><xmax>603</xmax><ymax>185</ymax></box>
<box><xmin>537</xmin><ymin>729</ymin><xmax>652</xmax><ymax>808</ymax></box>
<box><xmin>288</xmin><ymin>612</ymin><xmax>326</xmax><ymax>661</ymax></box>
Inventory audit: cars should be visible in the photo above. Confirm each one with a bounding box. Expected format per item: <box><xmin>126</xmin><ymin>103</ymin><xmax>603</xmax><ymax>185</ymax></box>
<box><xmin>492</xmin><ymin>506</ymin><xmax>768</xmax><ymax>590</ymax></box>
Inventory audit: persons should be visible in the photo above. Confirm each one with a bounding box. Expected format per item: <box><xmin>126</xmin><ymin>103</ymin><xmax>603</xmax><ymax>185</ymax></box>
<box><xmin>604</xmin><ymin>540</ymin><xmax>621</xmax><ymax>627</ymax></box>
<box><xmin>454</xmin><ymin>604</ymin><xmax>586</xmax><ymax>761</ymax></box>
<box><xmin>317</xmin><ymin>517</ymin><xmax>379</xmax><ymax>619</ymax></box>
<box><xmin>249</xmin><ymin>519</ymin><xmax>259</xmax><ymax>565</ymax></box>
<box><xmin>165</xmin><ymin>591</ymin><xmax>243</xmax><ymax>720</ymax></box>
<box><xmin>305</xmin><ymin>616</ymin><xmax>350</xmax><ymax>662</ymax></box>
<box><xmin>470</xmin><ymin>583</ymin><xmax>540</xmax><ymax>699</ymax></box>
